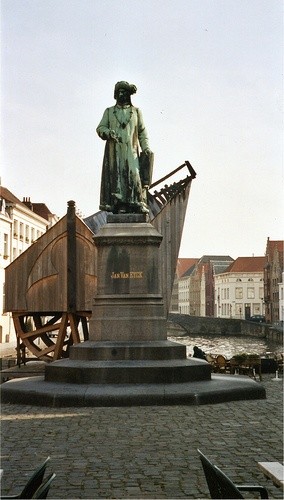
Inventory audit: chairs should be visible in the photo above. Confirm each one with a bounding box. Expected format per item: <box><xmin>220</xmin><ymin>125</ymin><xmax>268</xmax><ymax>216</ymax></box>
<box><xmin>197</xmin><ymin>449</ymin><xmax>268</xmax><ymax>499</ymax></box>
<box><xmin>0</xmin><ymin>456</ymin><xmax>56</xmax><ymax>499</ymax></box>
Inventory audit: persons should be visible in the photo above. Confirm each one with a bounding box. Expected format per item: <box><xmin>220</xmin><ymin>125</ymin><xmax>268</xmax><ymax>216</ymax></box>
<box><xmin>96</xmin><ymin>80</ymin><xmax>153</xmax><ymax>215</ymax></box>
<box><xmin>192</xmin><ymin>345</ymin><xmax>208</xmax><ymax>362</ymax></box>
